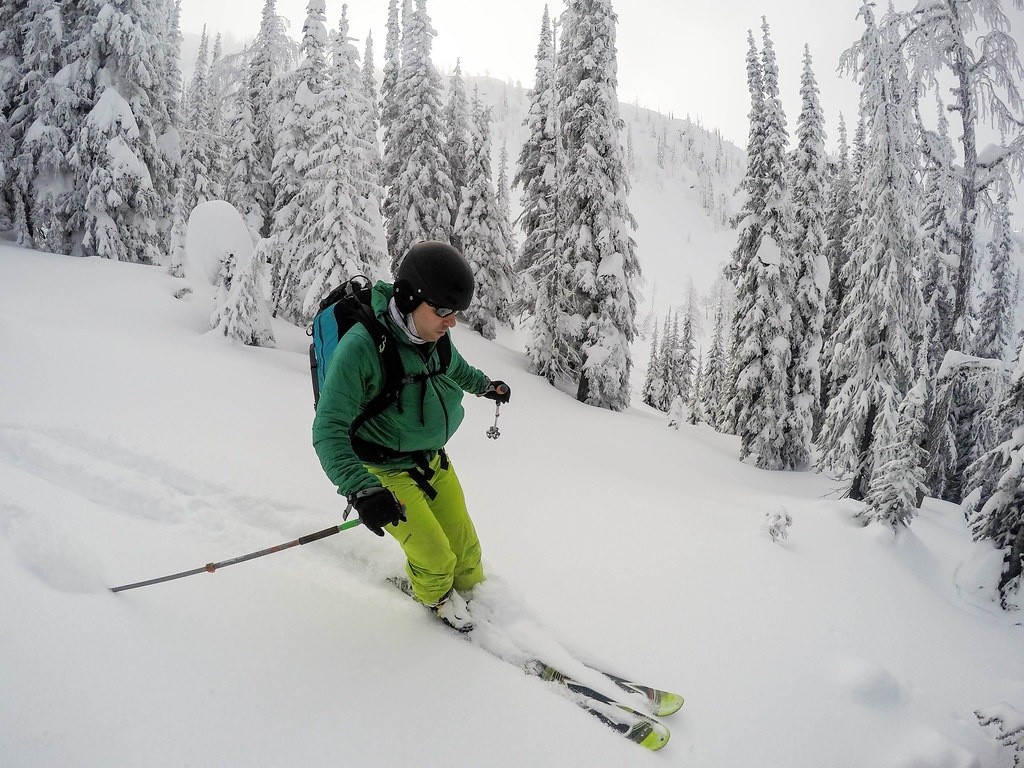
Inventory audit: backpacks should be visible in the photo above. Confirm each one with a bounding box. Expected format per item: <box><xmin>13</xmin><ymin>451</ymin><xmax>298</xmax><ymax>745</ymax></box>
<box><xmin>305</xmin><ymin>274</ymin><xmax>452</xmax><ymax>441</ymax></box>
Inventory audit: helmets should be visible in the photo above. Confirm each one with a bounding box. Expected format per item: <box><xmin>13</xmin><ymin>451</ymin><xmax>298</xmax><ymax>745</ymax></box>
<box><xmin>396</xmin><ymin>241</ymin><xmax>475</xmax><ymax>311</ymax></box>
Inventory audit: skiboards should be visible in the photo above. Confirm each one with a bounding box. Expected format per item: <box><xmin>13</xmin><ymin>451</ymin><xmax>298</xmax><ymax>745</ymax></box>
<box><xmin>386</xmin><ymin>571</ymin><xmax>685</xmax><ymax>752</ymax></box>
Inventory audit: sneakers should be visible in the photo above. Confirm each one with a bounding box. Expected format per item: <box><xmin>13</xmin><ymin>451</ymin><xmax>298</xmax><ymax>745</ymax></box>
<box><xmin>430</xmin><ymin>587</ymin><xmax>493</xmax><ymax>632</ymax></box>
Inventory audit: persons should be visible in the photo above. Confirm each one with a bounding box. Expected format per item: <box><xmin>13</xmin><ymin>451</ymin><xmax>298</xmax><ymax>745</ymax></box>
<box><xmin>311</xmin><ymin>239</ymin><xmax>512</xmax><ymax>635</ymax></box>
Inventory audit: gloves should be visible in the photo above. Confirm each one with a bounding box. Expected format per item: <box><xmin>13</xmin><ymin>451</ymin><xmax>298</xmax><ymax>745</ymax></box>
<box><xmin>483</xmin><ymin>381</ymin><xmax>511</xmax><ymax>404</ymax></box>
<box><xmin>351</xmin><ymin>486</ymin><xmax>407</xmax><ymax>537</ymax></box>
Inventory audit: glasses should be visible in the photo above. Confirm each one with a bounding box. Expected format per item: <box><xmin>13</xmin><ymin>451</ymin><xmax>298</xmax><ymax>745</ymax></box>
<box><xmin>414</xmin><ymin>293</ymin><xmax>462</xmax><ymax>318</ymax></box>
